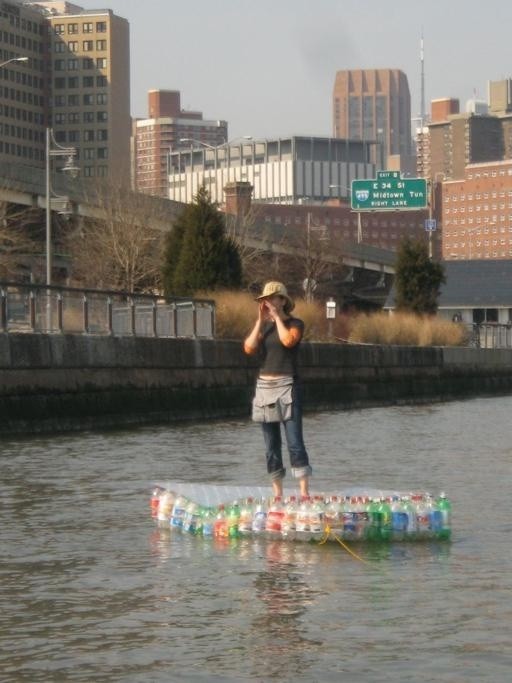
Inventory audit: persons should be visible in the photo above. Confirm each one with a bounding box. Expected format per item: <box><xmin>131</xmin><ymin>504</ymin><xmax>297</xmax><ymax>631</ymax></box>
<box><xmin>241</xmin><ymin>278</ymin><xmax>312</xmax><ymax>502</ymax></box>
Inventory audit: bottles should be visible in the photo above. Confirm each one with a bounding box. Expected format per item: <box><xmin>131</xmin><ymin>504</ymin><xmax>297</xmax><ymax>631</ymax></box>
<box><xmin>148</xmin><ymin>488</ymin><xmax>453</xmax><ymax>542</ymax></box>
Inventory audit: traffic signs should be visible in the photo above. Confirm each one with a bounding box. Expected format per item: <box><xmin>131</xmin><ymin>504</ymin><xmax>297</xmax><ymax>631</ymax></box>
<box><xmin>350</xmin><ymin>169</ymin><xmax>428</xmax><ymax>213</ymax></box>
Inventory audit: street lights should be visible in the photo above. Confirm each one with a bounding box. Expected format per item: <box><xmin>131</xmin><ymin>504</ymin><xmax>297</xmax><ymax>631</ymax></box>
<box><xmin>1</xmin><ymin>56</ymin><xmax>29</xmax><ymax>66</ymax></box>
<box><xmin>329</xmin><ymin>184</ymin><xmax>363</xmax><ymax>244</ymax></box>
<box><xmin>306</xmin><ymin>211</ymin><xmax>332</xmax><ymax>304</ymax></box>
<box><xmin>46</xmin><ymin>127</ymin><xmax>81</xmax><ymax>332</ymax></box>
<box><xmin>179</xmin><ymin>135</ymin><xmax>253</xmax><ymax>208</ymax></box>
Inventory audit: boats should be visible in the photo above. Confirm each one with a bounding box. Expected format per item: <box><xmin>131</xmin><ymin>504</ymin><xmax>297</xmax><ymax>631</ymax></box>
<box><xmin>151</xmin><ymin>478</ymin><xmax>452</xmax><ymax>544</ymax></box>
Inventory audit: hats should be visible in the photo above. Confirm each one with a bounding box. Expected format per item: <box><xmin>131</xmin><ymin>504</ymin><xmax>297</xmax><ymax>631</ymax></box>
<box><xmin>254</xmin><ymin>281</ymin><xmax>295</xmax><ymax>312</ymax></box>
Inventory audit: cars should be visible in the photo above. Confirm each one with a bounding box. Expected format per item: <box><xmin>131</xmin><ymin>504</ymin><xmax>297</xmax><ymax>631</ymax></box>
<box><xmin>1</xmin><ymin>290</ymin><xmax>58</xmax><ymax>323</ymax></box>
